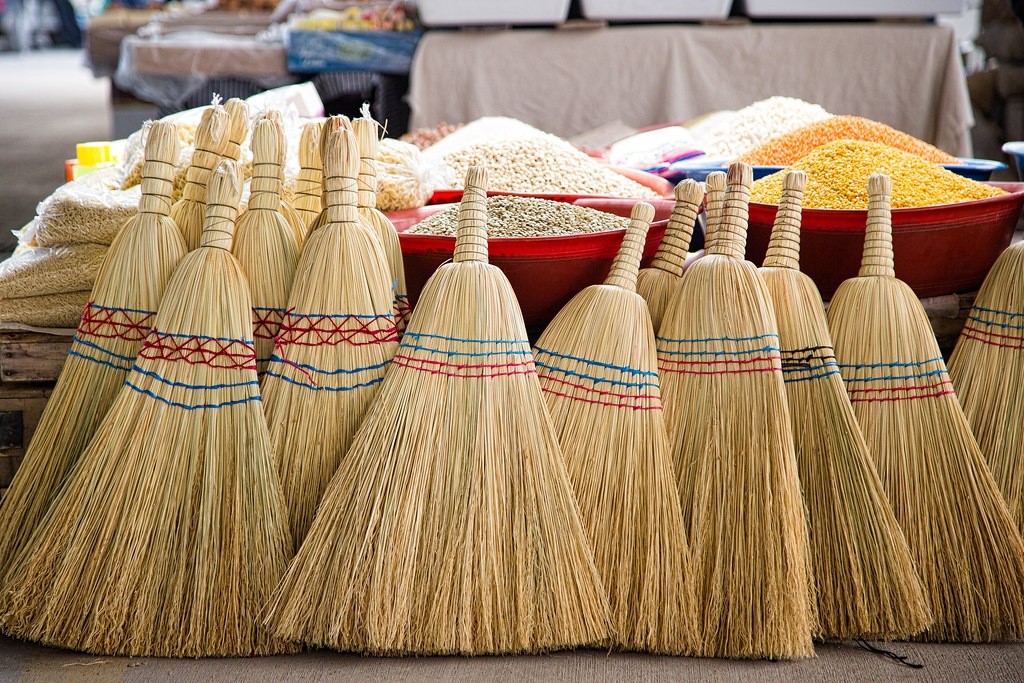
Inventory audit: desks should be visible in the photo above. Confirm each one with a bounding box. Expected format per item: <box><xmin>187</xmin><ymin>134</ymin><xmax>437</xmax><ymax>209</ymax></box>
<box><xmin>403</xmin><ymin>27</ymin><xmax>975</xmax><ymax>161</ymax></box>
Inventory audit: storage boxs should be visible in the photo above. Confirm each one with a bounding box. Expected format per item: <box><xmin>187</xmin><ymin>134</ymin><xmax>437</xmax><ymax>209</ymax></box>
<box><xmin>746</xmin><ymin>0</ymin><xmax>966</xmax><ymax>17</ymax></box>
<box><xmin>127</xmin><ymin>19</ymin><xmax>287</xmax><ymax>79</ymax></box>
<box><xmin>582</xmin><ymin>0</ymin><xmax>733</xmax><ymax>20</ymax></box>
<box><xmin>288</xmin><ymin>7</ymin><xmax>425</xmax><ymax>71</ymax></box>
<box><xmin>86</xmin><ymin>6</ymin><xmax>203</xmax><ymax>73</ymax></box>
<box><xmin>418</xmin><ymin>0</ymin><xmax>570</xmax><ymax>26</ymax></box>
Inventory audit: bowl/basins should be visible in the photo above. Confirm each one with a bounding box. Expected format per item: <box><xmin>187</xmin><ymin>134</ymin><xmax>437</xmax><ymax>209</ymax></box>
<box><xmin>381</xmin><ymin>198</ymin><xmax>702</xmax><ymax>322</ymax></box>
<box><xmin>409</xmin><ymin>164</ymin><xmax>675</xmax><ymax>205</ymax></box>
<box><xmin>703</xmin><ymin>181</ymin><xmax>1024</xmax><ymax>299</ymax></box>
<box><xmin>671</xmin><ymin>157</ymin><xmax>1010</xmax><ymax>181</ymax></box>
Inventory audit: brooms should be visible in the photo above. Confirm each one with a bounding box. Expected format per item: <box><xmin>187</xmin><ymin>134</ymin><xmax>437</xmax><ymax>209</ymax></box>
<box><xmin>1</xmin><ymin>94</ymin><xmax>1023</xmax><ymax>666</ymax></box>
